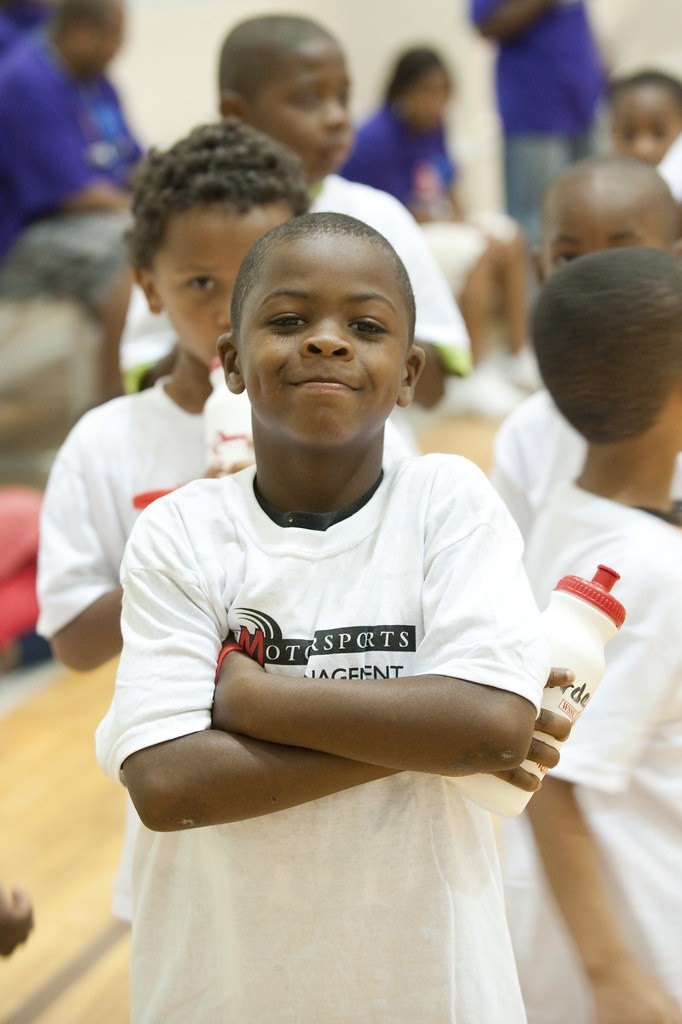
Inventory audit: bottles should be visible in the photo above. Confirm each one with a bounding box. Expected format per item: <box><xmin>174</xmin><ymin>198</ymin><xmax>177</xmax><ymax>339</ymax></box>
<box><xmin>201</xmin><ymin>354</ymin><xmax>254</xmax><ymax>473</ymax></box>
<box><xmin>457</xmin><ymin>565</ymin><xmax>625</xmax><ymax>820</ymax></box>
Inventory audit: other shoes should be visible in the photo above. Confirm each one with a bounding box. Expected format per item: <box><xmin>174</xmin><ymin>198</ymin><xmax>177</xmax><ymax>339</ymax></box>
<box><xmin>474</xmin><ymin>356</ymin><xmax>512</xmax><ymax>385</ymax></box>
<box><xmin>511</xmin><ymin>348</ymin><xmax>544</xmax><ymax>391</ymax></box>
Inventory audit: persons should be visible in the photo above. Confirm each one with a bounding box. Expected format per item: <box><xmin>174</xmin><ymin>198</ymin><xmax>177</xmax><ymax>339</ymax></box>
<box><xmin>329</xmin><ymin>41</ymin><xmax>545</xmax><ymax>397</ymax></box>
<box><xmin>606</xmin><ymin>73</ymin><xmax>681</xmax><ymax>206</ymax></box>
<box><xmin>37</xmin><ymin>121</ymin><xmax>312</xmax><ymax>671</ymax></box>
<box><xmin>91</xmin><ymin>212</ymin><xmax>575</xmax><ymax>1024</ymax></box>
<box><xmin>490</xmin><ymin>154</ymin><xmax>682</xmax><ymax>545</ymax></box>
<box><xmin>0</xmin><ymin>880</ymin><xmax>34</xmax><ymax>956</ymax></box>
<box><xmin>494</xmin><ymin>247</ymin><xmax>682</xmax><ymax>1023</ymax></box>
<box><xmin>470</xmin><ymin>0</ymin><xmax>610</xmax><ymax>246</ymax></box>
<box><xmin>119</xmin><ymin>15</ymin><xmax>472</xmax><ymax>468</ymax></box>
<box><xmin>1</xmin><ymin>0</ymin><xmax>144</xmax><ymax>403</ymax></box>
